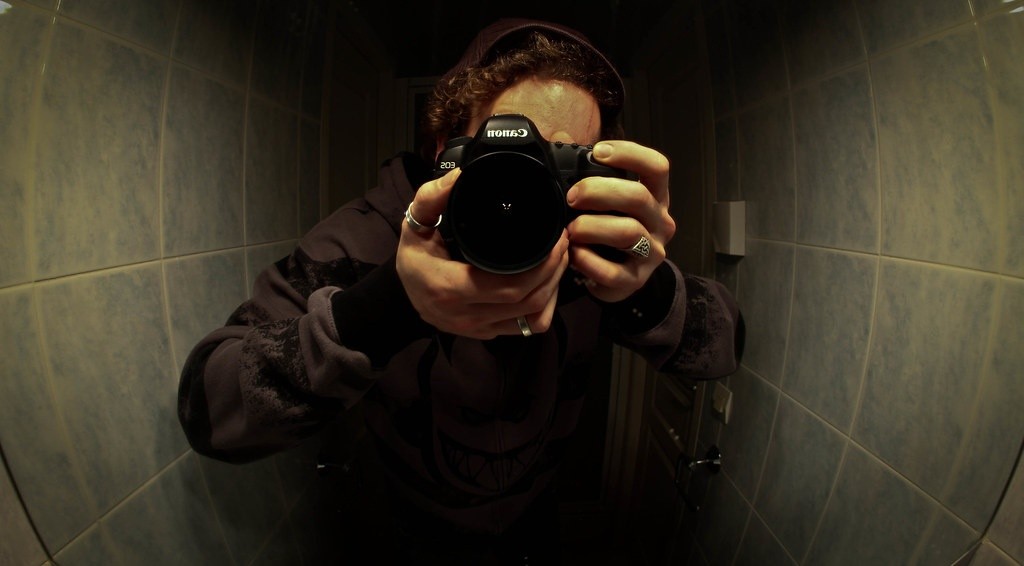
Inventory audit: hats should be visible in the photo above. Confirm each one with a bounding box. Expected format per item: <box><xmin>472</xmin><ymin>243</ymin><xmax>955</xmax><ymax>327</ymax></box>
<box><xmin>442</xmin><ymin>18</ymin><xmax>626</xmax><ymax>137</ymax></box>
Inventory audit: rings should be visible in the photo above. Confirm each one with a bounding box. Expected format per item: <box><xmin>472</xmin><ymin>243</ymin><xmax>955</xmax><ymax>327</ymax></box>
<box><xmin>406</xmin><ymin>202</ymin><xmax>442</xmax><ymax>233</ymax></box>
<box><xmin>616</xmin><ymin>237</ymin><xmax>651</xmax><ymax>260</ymax></box>
<box><xmin>516</xmin><ymin>315</ymin><xmax>532</xmax><ymax>337</ymax></box>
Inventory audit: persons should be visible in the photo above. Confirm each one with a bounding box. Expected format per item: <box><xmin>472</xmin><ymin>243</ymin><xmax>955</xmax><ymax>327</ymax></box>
<box><xmin>176</xmin><ymin>17</ymin><xmax>746</xmax><ymax>566</ymax></box>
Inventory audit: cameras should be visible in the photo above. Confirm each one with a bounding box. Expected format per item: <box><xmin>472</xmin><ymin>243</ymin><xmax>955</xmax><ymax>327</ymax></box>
<box><xmin>436</xmin><ymin>114</ymin><xmax>640</xmax><ymax>307</ymax></box>
